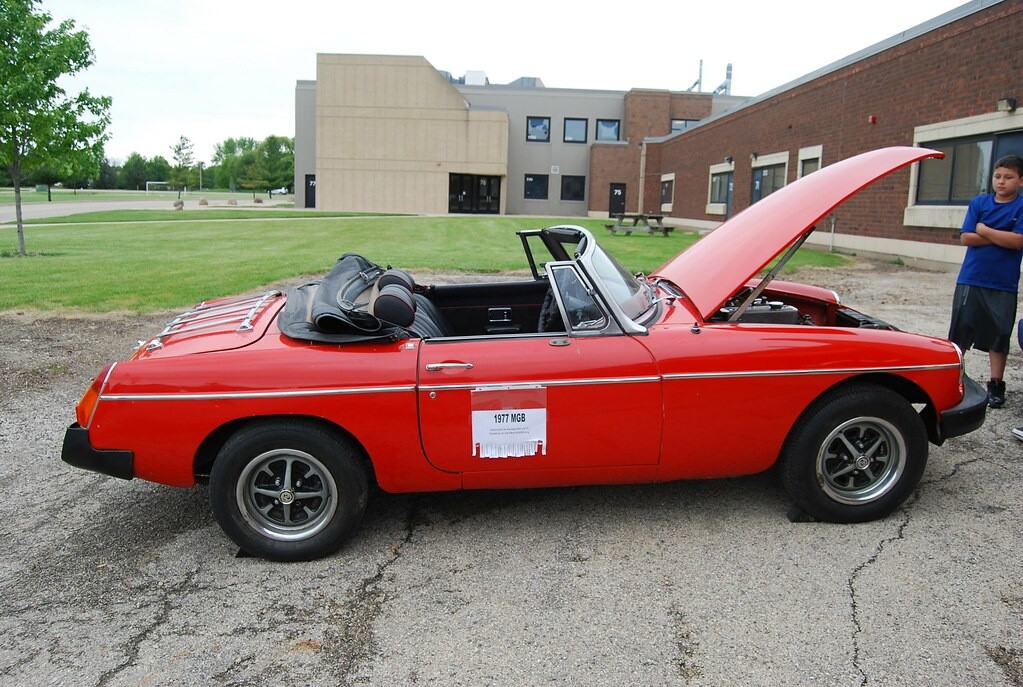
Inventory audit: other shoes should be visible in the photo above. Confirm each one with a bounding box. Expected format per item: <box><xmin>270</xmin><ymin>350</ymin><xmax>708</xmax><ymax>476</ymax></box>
<box><xmin>1011</xmin><ymin>427</ymin><xmax>1023</xmax><ymax>442</ymax></box>
<box><xmin>987</xmin><ymin>380</ymin><xmax>1006</xmax><ymax>409</ymax></box>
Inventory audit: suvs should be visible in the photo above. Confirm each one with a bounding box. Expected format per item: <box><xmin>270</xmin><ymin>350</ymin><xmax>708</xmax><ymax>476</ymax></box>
<box><xmin>268</xmin><ymin>186</ymin><xmax>289</xmax><ymax>196</ymax></box>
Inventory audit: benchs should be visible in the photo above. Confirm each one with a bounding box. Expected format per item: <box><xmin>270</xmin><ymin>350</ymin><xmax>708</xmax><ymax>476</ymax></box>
<box><xmin>605</xmin><ymin>224</ymin><xmax>673</xmax><ymax>232</ymax></box>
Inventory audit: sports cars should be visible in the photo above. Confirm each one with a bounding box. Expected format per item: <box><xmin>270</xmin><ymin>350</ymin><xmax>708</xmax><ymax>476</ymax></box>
<box><xmin>59</xmin><ymin>146</ymin><xmax>992</xmax><ymax>564</ymax></box>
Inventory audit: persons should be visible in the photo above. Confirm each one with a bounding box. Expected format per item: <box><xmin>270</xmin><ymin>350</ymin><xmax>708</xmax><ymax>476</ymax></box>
<box><xmin>949</xmin><ymin>155</ymin><xmax>1023</xmax><ymax>440</ymax></box>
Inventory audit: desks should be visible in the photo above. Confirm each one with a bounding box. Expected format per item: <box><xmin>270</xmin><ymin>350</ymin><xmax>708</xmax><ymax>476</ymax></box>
<box><xmin>612</xmin><ymin>214</ymin><xmax>669</xmax><ymax>237</ymax></box>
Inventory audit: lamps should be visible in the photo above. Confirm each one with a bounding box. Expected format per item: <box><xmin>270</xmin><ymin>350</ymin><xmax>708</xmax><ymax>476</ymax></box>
<box><xmin>997</xmin><ymin>98</ymin><xmax>1017</xmax><ymax>112</ymax></box>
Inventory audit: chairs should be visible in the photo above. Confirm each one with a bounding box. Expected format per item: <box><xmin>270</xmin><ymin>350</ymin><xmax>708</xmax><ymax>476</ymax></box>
<box><xmin>374</xmin><ymin>269</ymin><xmax>455</xmax><ymax>339</ymax></box>
